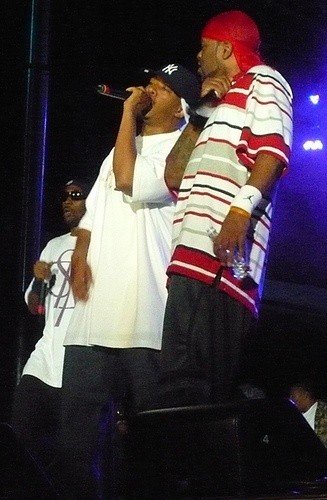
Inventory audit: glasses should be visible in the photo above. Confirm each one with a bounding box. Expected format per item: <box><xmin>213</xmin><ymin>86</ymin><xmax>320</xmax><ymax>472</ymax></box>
<box><xmin>63</xmin><ymin>192</ymin><xmax>86</xmax><ymax>202</ymax></box>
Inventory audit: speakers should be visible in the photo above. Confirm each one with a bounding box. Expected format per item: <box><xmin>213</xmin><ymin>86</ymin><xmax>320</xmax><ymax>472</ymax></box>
<box><xmin>126</xmin><ymin>397</ymin><xmax>327</xmax><ymax>500</ymax></box>
<box><xmin>0</xmin><ymin>423</ymin><xmax>63</xmax><ymax>500</ymax></box>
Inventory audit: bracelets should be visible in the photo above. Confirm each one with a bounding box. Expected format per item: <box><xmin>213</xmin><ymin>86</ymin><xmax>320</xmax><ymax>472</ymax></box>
<box><xmin>187</xmin><ymin>112</ymin><xmax>210</xmax><ymax>130</ymax></box>
<box><xmin>230</xmin><ymin>207</ymin><xmax>252</xmax><ymax>220</ymax></box>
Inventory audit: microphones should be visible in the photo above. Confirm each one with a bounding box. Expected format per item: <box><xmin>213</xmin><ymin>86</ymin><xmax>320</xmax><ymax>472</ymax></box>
<box><xmin>187</xmin><ymin>89</ymin><xmax>223</xmax><ymax>115</ymax></box>
<box><xmin>35</xmin><ymin>277</ymin><xmax>48</xmax><ymax>314</ymax></box>
<box><xmin>96</xmin><ymin>84</ymin><xmax>132</xmax><ymax>100</ymax></box>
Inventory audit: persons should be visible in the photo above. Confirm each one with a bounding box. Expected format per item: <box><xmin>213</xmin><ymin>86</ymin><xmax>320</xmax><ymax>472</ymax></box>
<box><xmin>8</xmin><ymin>177</ymin><xmax>94</xmax><ymax>499</ymax></box>
<box><xmin>161</xmin><ymin>10</ymin><xmax>294</xmax><ymax>414</ymax></box>
<box><xmin>59</xmin><ymin>62</ymin><xmax>200</xmax><ymax>500</ymax></box>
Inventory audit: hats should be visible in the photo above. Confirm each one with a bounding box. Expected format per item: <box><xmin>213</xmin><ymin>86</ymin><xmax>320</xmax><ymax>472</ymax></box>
<box><xmin>65</xmin><ymin>176</ymin><xmax>92</xmax><ymax>198</ymax></box>
<box><xmin>201</xmin><ymin>10</ymin><xmax>267</xmax><ymax>74</ymax></box>
<box><xmin>158</xmin><ymin>63</ymin><xmax>199</xmax><ymax>110</ymax></box>
